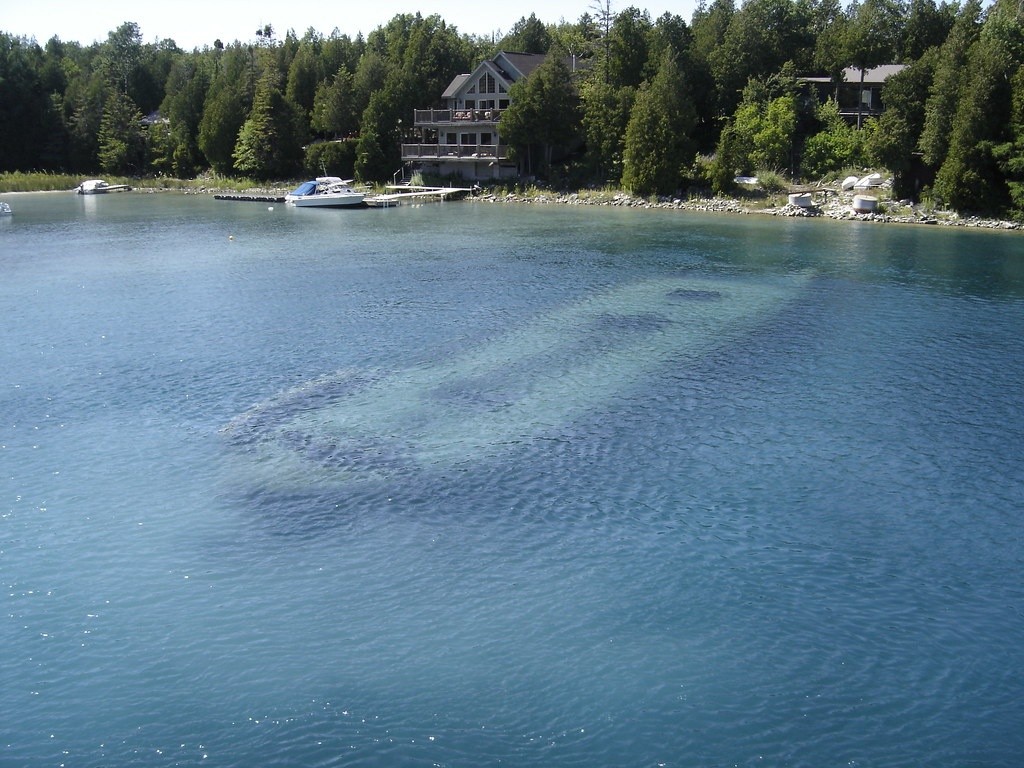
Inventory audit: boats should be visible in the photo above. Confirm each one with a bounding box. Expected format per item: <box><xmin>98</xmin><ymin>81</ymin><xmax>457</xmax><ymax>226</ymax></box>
<box><xmin>285</xmin><ymin>179</ymin><xmax>365</xmax><ymax>207</ymax></box>
<box><xmin>73</xmin><ymin>179</ymin><xmax>131</xmax><ymax>194</ymax></box>
